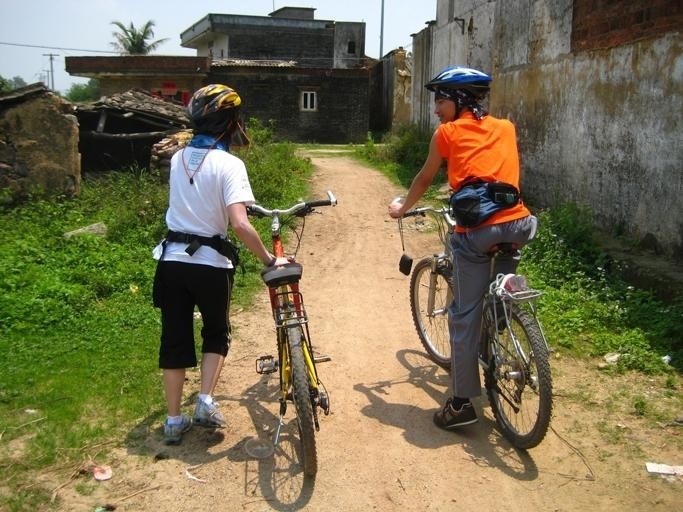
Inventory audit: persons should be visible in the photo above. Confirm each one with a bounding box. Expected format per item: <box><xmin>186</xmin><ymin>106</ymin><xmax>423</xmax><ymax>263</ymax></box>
<box><xmin>151</xmin><ymin>83</ymin><xmax>291</xmax><ymax>446</ymax></box>
<box><xmin>387</xmin><ymin>66</ymin><xmax>537</xmax><ymax>430</ymax></box>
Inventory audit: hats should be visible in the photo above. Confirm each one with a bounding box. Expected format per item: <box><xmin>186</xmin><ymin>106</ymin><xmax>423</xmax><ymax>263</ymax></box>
<box><xmin>184</xmin><ymin>83</ymin><xmax>243</xmax><ymax>133</ymax></box>
<box><xmin>425</xmin><ymin>66</ymin><xmax>492</xmax><ymax>93</ymax></box>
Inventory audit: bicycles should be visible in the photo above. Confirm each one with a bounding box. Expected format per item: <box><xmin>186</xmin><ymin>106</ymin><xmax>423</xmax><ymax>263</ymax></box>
<box><xmin>389</xmin><ymin>192</ymin><xmax>553</xmax><ymax>450</ymax></box>
<box><xmin>244</xmin><ymin>188</ymin><xmax>338</xmax><ymax>480</ymax></box>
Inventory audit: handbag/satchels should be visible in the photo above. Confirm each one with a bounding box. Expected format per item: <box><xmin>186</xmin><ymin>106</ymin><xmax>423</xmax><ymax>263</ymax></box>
<box><xmin>164</xmin><ymin>230</ymin><xmax>241</xmax><ymax>275</ymax></box>
<box><xmin>451</xmin><ymin>179</ymin><xmax>520</xmax><ymax>230</ymax></box>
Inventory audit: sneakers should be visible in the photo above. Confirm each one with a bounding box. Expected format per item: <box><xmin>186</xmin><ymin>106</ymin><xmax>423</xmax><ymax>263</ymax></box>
<box><xmin>192</xmin><ymin>396</ymin><xmax>227</xmax><ymax>429</ymax></box>
<box><xmin>432</xmin><ymin>401</ymin><xmax>479</xmax><ymax>430</ymax></box>
<box><xmin>164</xmin><ymin>415</ymin><xmax>193</xmax><ymax>445</ymax></box>
